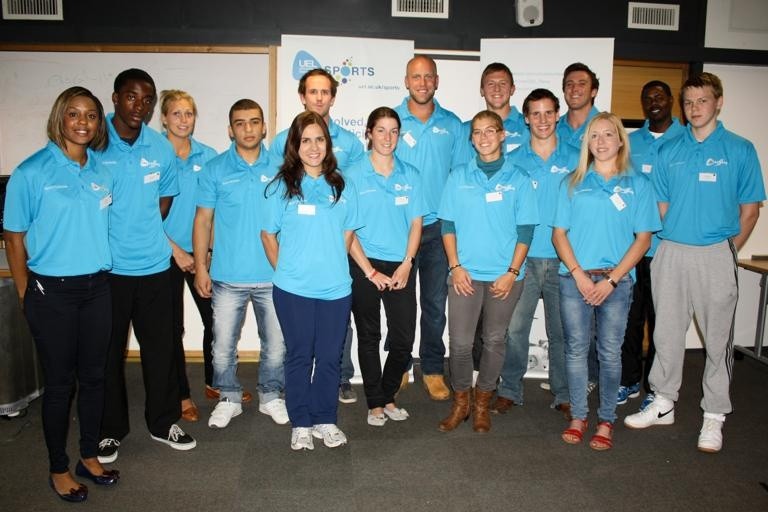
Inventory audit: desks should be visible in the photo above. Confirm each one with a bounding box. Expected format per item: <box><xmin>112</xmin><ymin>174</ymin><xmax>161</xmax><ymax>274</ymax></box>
<box><xmin>735</xmin><ymin>259</ymin><xmax>768</xmax><ymax>364</ymax></box>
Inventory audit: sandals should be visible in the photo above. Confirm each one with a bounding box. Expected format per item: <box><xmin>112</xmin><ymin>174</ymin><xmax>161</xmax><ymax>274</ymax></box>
<box><xmin>562</xmin><ymin>416</ymin><xmax>589</xmax><ymax>444</ymax></box>
<box><xmin>588</xmin><ymin>422</ymin><xmax>614</xmax><ymax>450</ymax></box>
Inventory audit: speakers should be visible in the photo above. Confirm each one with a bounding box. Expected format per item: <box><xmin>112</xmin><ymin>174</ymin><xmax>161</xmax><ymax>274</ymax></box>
<box><xmin>515</xmin><ymin>1</ymin><xmax>543</xmax><ymax>27</ymax></box>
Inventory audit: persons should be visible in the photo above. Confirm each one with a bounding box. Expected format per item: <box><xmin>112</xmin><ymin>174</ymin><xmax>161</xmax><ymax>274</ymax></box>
<box><xmin>93</xmin><ymin>57</ymin><xmax>767</xmax><ymax>465</ymax></box>
<box><xmin>0</xmin><ymin>84</ymin><xmax>122</xmax><ymax>503</ymax></box>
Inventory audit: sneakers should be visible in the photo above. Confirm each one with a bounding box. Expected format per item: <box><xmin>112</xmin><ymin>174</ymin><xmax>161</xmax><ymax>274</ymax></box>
<box><xmin>47</xmin><ymin>439</ymin><xmax>122</xmax><ymax>504</ymax></box>
<box><xmin>150</xmin><ymin>380</ymin><xmax>252</xmax><ymax>452</ymax></box>
<box><xmin>258</xmin><ymin>375</ymin><xmax>409</xmax><ymax>450</ymax></box>
<box><xmin>617</xmin><ymin>383</ymin><xmax>677</xmax><ymax>428</ymax></box>
<box><xmin>698</xmin><ymin>417</ymin><xmax>728</xmax><ymax>453</ymax></box>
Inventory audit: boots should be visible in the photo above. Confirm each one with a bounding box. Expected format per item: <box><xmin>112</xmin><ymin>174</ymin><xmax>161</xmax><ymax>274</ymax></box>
<box><xmin>437</xmin><ymin>388</ymin><xmax>471</xmax><ymax>433</ymax></box>
<box><xmin>473</xmin><ymin>384</ymin><xmax>493</xmax><ymax>434</ymax></box>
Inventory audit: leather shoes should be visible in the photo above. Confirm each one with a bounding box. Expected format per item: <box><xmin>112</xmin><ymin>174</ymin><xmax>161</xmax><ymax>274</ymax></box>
<box><xmin>422</xmin><ymin>374</ymin><xmax>451</xmax><ymax>402</ymax></box>
<box><xmin>585</xmin><ymin>269</ymin><xmax>613</xmax><ymax>275</ymax></box>
<box><xmin>557</xmin><ymin>400</ymin><xmax>573</xmax><ymax>421</ymax></box>
<box><xmin>486</xmin><ymin>395</ymin><xmax>514</xmax><ymax>414</ymax></box>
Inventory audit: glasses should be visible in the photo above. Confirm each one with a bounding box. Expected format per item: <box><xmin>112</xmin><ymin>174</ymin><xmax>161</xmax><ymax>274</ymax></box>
<box><xmin>527</xmin><ymin>110</ymin><xmax>555</xmax><ymax>119</ymax></box>
<box><xmin>471</xmin><ymin>127</ymin><xmax>502</xmax><ymax>138</ymax></box>
